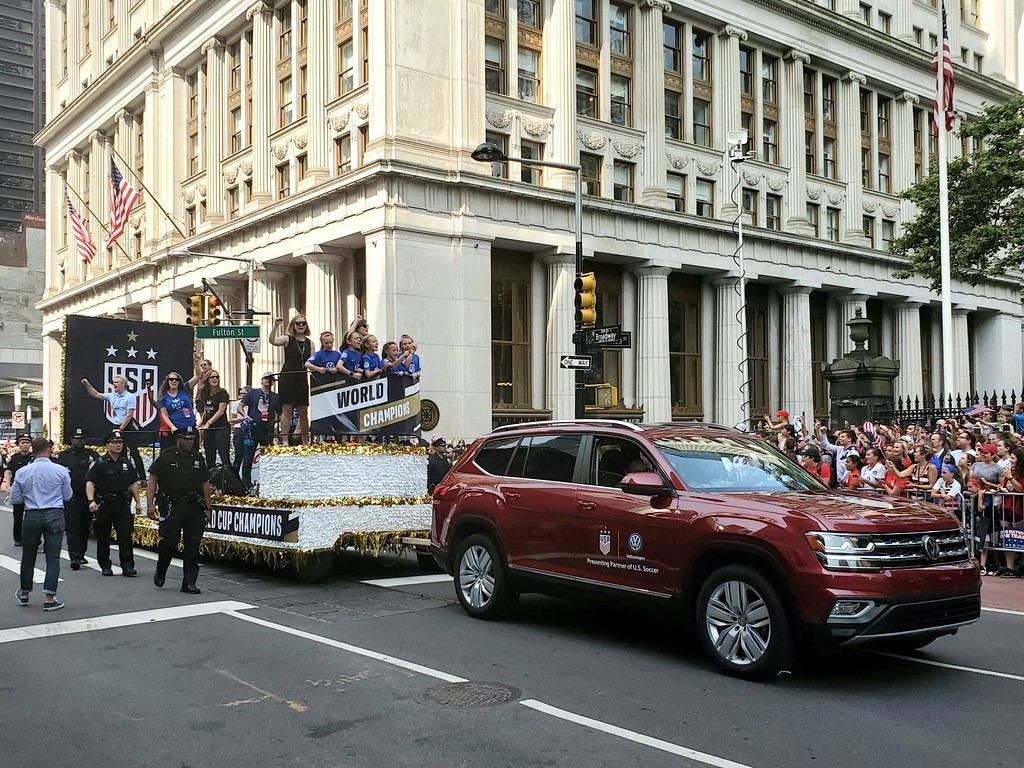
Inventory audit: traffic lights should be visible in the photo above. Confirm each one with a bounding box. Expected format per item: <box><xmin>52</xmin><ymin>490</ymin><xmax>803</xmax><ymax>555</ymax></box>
<box><xmin>574</xmin><ymin>272</ymin><xmax>597</xmax><ymax>325</ymax></box>
<box><xmin>560</xmin><ymin>355</ymin><xmax>592</xmax><ymax>372</ymax></box>
<box><xmin>208</xmin><ymin>297</ymin><xmax>221</xmax><ymax>327</ymax></box>
<box><xmin>186</xmin><ymin>296</ymin><xmax>202</xmax><ymax>326</ymax></box>
<box><xmin>595</xmin><ymin>386</ymin><xmax>617</xmax><ymax>408</ymax></box>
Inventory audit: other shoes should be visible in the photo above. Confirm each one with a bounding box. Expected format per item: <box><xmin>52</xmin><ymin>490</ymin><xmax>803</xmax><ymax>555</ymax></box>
<box><xmin>70</xmin><ymin>559</ymin><xmax>87</xmax><ymax>570</ymax></box>
<box><xmin>181</xmin><ymin>584</ymin><xmax>201</xmax><ymax>594</ymax></box>
<box><xmin>14</xmin><ymin>541</ymin><xmax>22</xmax><ymax>546</ymax></box>
<box><xmin>981</xmin><ymin>565</ymin><xmax>1024</xmax><ymax>579</ymax></box>
<box><xmin>122</xmin><ymin>568</ymin><xmax>137</xmax><ymax>576</ymax></box>
<box><xmin>102</xmin><ymin>568</ymin><xmax>113</xmax><ymax>576</ymax></box>
<box><xmin>154</xmin><ymin>571</ymin><xmax>165</xmax><ymax>587</ymax></box>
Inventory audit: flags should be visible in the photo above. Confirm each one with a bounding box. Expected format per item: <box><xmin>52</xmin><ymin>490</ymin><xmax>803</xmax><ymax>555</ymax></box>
<box><xmin>858</xmin><ymin>421</ymin><xmax>884</xmax><ymax>450</ymax></box>
<box><xmin>66</xmin><ymin>192</ymin><xmax>97</xmax><ymax>265</ymax></box>
<box><xmin>932</xmin><ymin>1</ymin><xmax>954</xmax><ymax>139</ymax></box>
<box><xmin>107</xmin><ymin>156</ymin><xmax>141</xmax><ymax>248</ymax></box>
<box><xmin>962</xmin><ymin>404</ymin><xmax>992</xmax><ymax>418</ymax></box>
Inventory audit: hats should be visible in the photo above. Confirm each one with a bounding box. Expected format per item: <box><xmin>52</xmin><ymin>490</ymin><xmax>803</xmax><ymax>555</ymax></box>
<box><xmin>801</xmin><ymin>448</ymin><xmax>820</xmax><ymax>459</ymax></box>
<box><xmin>978</xmin><ymin>443</ymin><xmax>997</xmax><ymax>454</ymax></box>
<box><xmin>16</xmin><ymin>434</ymin><xmax>31</xmax><ymax>443</ymax></box>
<box><xmin>775</xmin><ymin>410</ymin><xmax>788</xmax><ymax>418</ymax></box>
<box><xmin>431</xmin><ymin>438</ymin><xmax>447</xmax><ymax>446</ymax></box>
<box><xmin>175</xmin><ymin>425</ymin><xmax>199</xmax><ymax>438</ymax></box>
<box><xmin>262</xmin><ymin>372</ymin><xmax>273</xmax><ymax>380</ymax></box>
<box><xmin>104</xmin><ymin>431</ymin><xmax>123</xmax><ymax>446</ymax></box>
<box><xmin>70</xmin><ymin>428</ymin><xmax>89</xmax><ymax>439</ymax></box>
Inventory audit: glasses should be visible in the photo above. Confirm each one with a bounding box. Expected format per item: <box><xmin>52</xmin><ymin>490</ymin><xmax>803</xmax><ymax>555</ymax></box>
<box><xmin>200</xmin><ymin>364</ymin><xmax>206</xmax><ymax>367</ymax></box>
<box><xmin>296</xmin><ymin>321</ymin><xmax>306</xmax><ymax>326</ymax></box>
<box><xmin>210</xmin><ymin>376</ymin><xmax>219</xmax><ymax>379</ymax></box>
<box><xmin>169</xmin><ymin>378</ymin><xmax>179</xmax><ymax>381</ymax></box>
<box><xmin>363</xmin><ymin>325</ymin><xmax>369</xmax><ymax>328</ymax></box>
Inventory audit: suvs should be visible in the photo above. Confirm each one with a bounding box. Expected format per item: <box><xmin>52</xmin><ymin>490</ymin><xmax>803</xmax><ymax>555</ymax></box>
<box><xmin>429</xmin><ymin>419</ymin><xmax>983</xmax><ymax>683</ymax></box>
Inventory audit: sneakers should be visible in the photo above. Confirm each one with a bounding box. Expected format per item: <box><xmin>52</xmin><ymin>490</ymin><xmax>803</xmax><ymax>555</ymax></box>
<box><xmin>43</xmin><ymin>600</ymin><xmax>64</xmax><ymax>611</ymax></box>
<box><xmin>15</xmin><ymin>589</ymin><xmax>29</xmax><ymax>605</ymax></box>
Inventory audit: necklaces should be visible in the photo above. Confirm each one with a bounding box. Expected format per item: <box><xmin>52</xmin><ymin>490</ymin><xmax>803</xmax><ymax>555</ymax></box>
<box><xmin>295</xmin><ymin>336</ymin><xmax>306</xmax><ymax>369</ymax></box>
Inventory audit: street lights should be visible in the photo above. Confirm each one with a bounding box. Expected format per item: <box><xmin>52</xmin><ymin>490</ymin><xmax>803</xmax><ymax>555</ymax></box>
<box><xmin>170</xmin><ymin>248</ymin><xmax>255</xmax><ymax>388</ymax></box>
<box><xmin>726</xmin><ymin>129</ymin><xmax>752</xmax><ymax>434</ymax></box>
<box><xmin>471</xmin><ymin>142</ymin><xmax>587</xmax><ymax>421</ymax></box>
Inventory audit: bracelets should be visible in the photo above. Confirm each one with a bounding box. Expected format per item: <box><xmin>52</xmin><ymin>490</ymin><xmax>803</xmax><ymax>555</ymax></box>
<box><xmin>87</xmin><ymin>499</ymin><xmax>95</xmax><ymax>504</ymax></box>
<box><xmin>204</xmin><ymin>506</ymin><xmax>212</xmax><ymax>511</ymax></box>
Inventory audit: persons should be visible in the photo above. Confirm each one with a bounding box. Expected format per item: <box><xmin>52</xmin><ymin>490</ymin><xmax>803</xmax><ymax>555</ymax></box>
<box><xmin>53</xmin><ymin>426</ymin><xmax>101</xmax><ymax>571</ymax></box>
<box><xmin>417</xmin><ymin>435</ymin><xmax>473</xmax><ymax>463</ymax></box>
<box><xmin>8</xmin><ymin>433</ymin><xmax>42</xmax><ymax>546</ymax></box>
<box><xmin>85</xmin><ymin>432</ymin><xmax>144</xmax><ymax>578</ymax></box>
<box><xmin>147</xmin><ymin>356</ymin><xmax>281</xmax><ymax>495</ymax></box>
<box><xmin>80</xmin><ymin>374</ymin><xmax>147</xmax><ymax>488</ymax></box>
<box><xmin>0</xmin><ymin>447</ymin><xmax>7</xmax><ymax>491</ymax></box>
<box><xmin>27</xmin><ymin>438</ymin><xmax>60</xmax><ymax>465</ymax></box>
<box><xmin>10</xmin><ymin>437</ymin><xmax>73</xmax><ymax>611</ymax></box>
<box><xmin>269</xmin><ymin>313</ymin><xmax>317</xmax><ymax>449</ymax></box>
<box><xmin>305</xmin><ymin>317</ymin><xmax>421</xmax><ymax>448</ymax></box>
<box><xmin>145</xmin><ymin>426</ymin><xmax>214</xmax><ymax>595</ymax></box>
<box><xmin>428</xmin><ymin>437</ymin><xmax>453</xmax><ymax>497</ymax></box>
<box><xmin>755</xmin><ymin>401</ymin><xmax>1024</xmax><ymax>576</ymax></box>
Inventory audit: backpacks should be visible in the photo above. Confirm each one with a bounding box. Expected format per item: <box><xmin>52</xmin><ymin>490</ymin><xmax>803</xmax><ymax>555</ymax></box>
<box><xmin>817</xmin><ymin>461</ymin><xmax>837</xmax><ymax>489</ymax></box>
<box><xmin>214</xmin><ymin>462</ymin><xmax>249</xmax><ymax>496</ymax></box>
